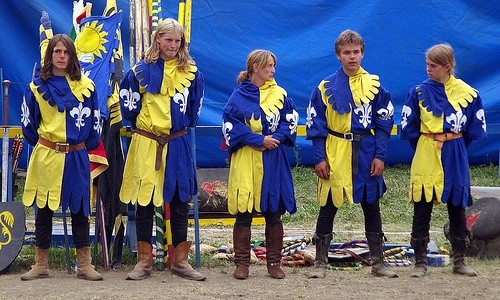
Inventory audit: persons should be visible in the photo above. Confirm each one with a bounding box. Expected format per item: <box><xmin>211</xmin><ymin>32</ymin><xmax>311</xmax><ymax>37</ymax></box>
<box><xmin>399</xmin><ymin>43</ymin><xmax>486</xmax><ymax>278</ymax></box>
<box><xmin>120</xmin><ymin>18</ymin><xmax>206</xmax><ymax>280</ymax></box>
<box><xmin>21</xmin><ymin>34</ymin><xmax>104</xmax><ymax>281</ymax></box>
<box><xmin>306</xmin><ymin>30</ymin><xmax>395</xmax><ymax>279</ymax></box>
<box><xmin>221</xmin><ymin>50</ymin><xmax>299</xmax><ymax>280</ymax></box>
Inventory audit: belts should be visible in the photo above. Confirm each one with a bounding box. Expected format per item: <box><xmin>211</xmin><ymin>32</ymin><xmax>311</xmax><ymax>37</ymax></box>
<box><xmin>327</xmin><ymin>128</ymin><xmax>374</xmax><ymax>176</ymax></box>
<box><xmin>422</xmin><ymin>130</ymin><xmax>463</xmax><ymax>148</ymax></box>
<box><xmin>37</xmin><ymin>135</ymin><xmax>85</xmax><ymax>154</ymax></box>
<box><xmin>135</xmin><ymin>127</ymin><xmax>188</xmax><ymax>171</ymax></box>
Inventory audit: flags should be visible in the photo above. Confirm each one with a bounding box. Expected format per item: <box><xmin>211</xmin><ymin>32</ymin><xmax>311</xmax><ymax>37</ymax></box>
<box><xmin>39</xmin><ymin>0</ymin><xmax>137</xmax><ymax>264</ymax></box>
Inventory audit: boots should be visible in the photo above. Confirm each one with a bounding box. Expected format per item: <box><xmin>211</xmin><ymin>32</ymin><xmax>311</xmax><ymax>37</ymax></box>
<box><xmin>170</xmin><ymin>241</ymin><xmax>206</xmax><ymax>282</ymax></box>
<box><xmin>264</xmin><ymin>222</ymin><xmax>286</xmax><ymax>279</ymax></box>
<box><xmin>20</xmin><ymin>245</ymin><xmax>50</xmax><ymax>281</ymax></box>
<box><xmin>306</xmin><ymin>232</ymin><xmax>334</xmax><ymax>279</ymax></box>
<box><xmin>365</xmin><ymin>231</ymin><xmax>399</xmax><ymax>278</ymax></box>
<box><xmin>448</xmin><ymin>233</ymin><xmax>478</xmax><ymax>276</ymax></box>
<box><xmin>409</xmin><ymin>232</ymin><xmax>430</xmax><ymax>278</ymax></box>
<box><xmin>232</xmin><ymin>222</ymin><xmax>251</xmax><ymax>279</ymax></box>
<box><xmin>125</xmin><ymin>241</ymin><xmax>153</xmax><ymax>281</ymax></box>
<box><xmin>75</xmin><ymin>246</ymin><xmax>104</xmax><ymax>281</ymax></box>
